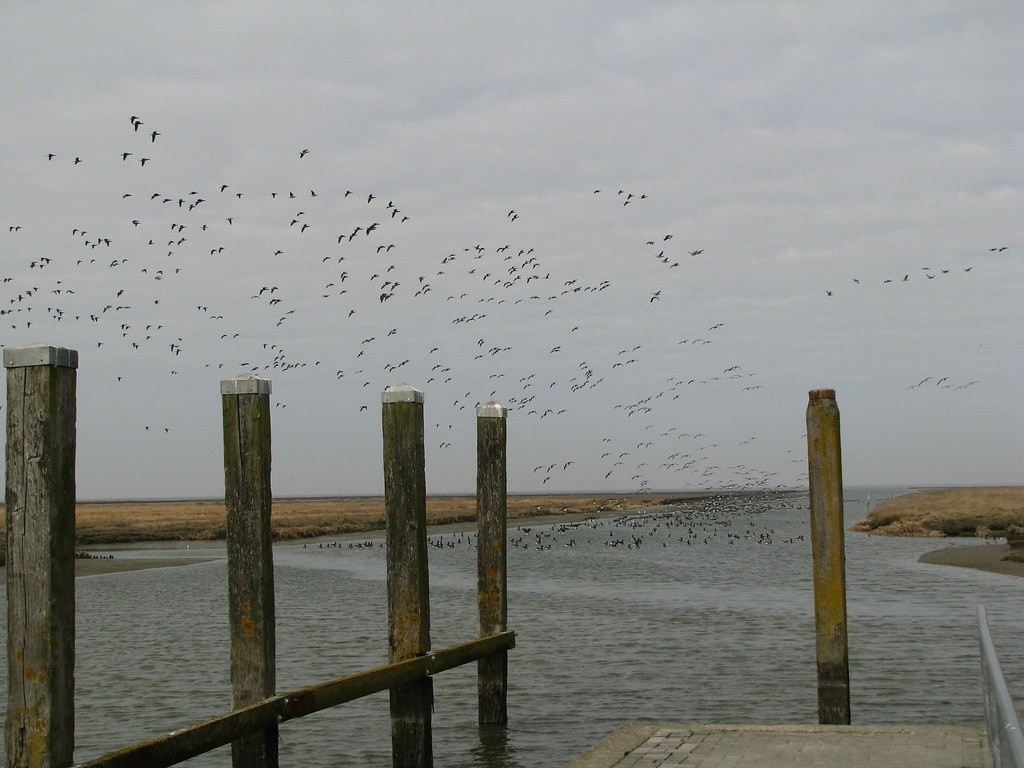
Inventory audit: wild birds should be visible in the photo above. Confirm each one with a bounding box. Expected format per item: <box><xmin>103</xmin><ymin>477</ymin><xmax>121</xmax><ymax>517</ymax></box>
<box><xmin>0</xmin><ymin>115</ymin><xmax>1011</xmax><ymax>553</ymax></box>
